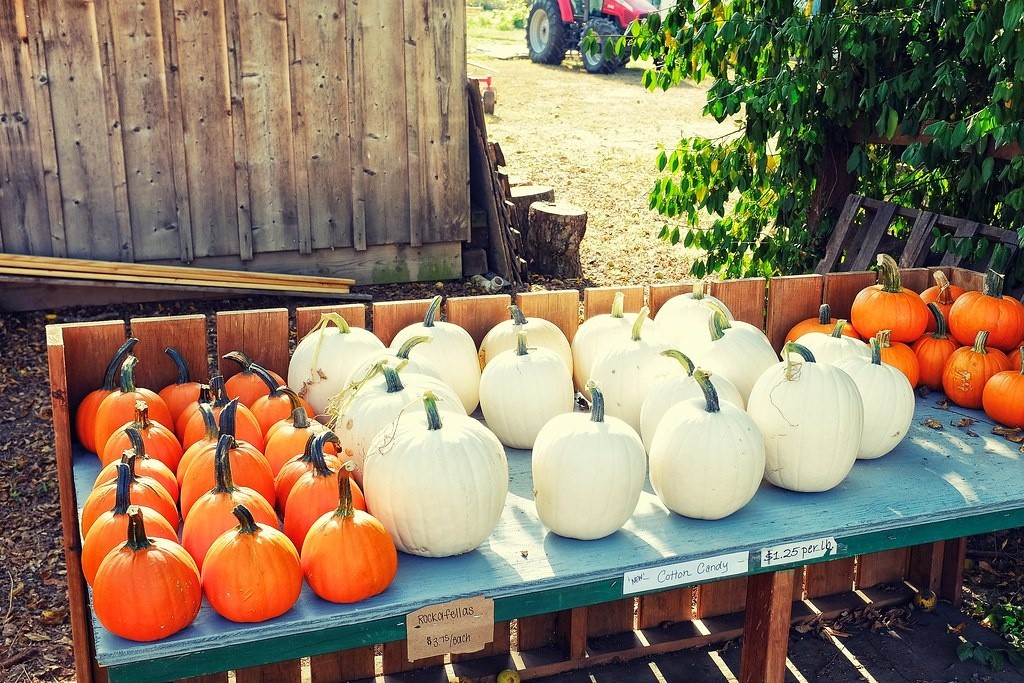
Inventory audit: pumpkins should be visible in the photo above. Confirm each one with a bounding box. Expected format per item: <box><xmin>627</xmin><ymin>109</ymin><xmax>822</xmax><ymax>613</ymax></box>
<box><xmin>77</xmin><ymin>337</ymin><xmax>399</xmax><ymax>643</ymax></box>
<box><xmin>286</xmin><ymin>280</ymin><xmax>916</xmax><ymax>557</ymax></box>
<box><xmin>783</xmin><ymin>253</ymin><xmax>1024</xmax><ymax>430</ymax></box>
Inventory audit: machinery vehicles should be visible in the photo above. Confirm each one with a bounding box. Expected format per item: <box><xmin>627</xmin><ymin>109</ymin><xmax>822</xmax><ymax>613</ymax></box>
<box><xmin>523</xmin><ymin>0</ymin><xmax>662</xmax><ymax>74</ymax></box>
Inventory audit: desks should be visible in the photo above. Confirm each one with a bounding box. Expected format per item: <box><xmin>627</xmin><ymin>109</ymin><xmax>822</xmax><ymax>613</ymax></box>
<box><xmin>46</xmin><ymin>266</ymin><xmax>1024</xmax><ymax>683</ymax></box>
